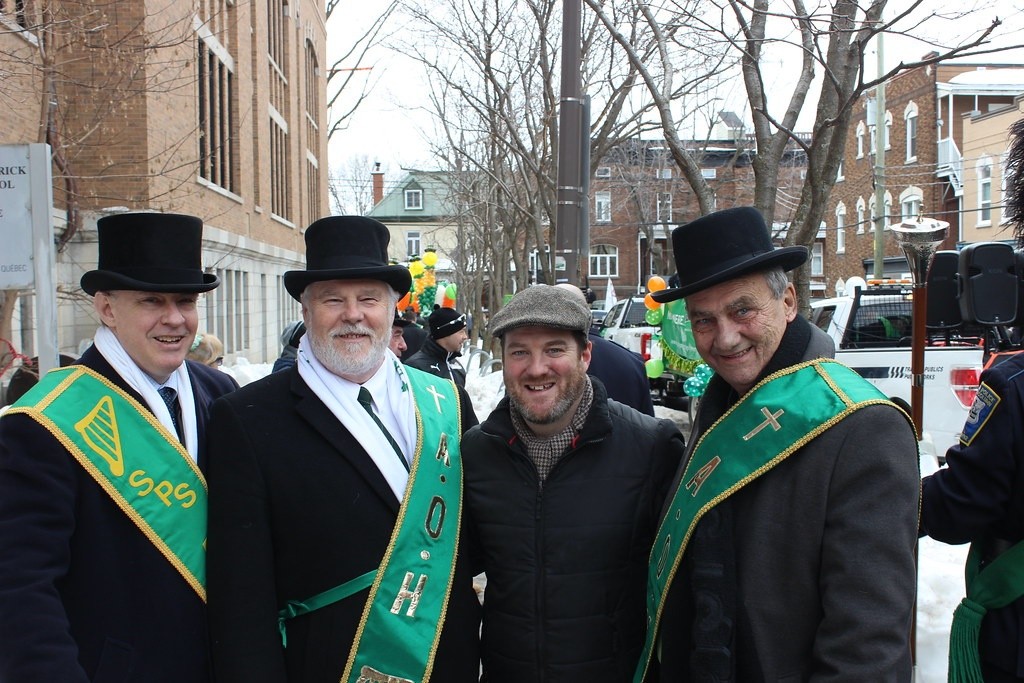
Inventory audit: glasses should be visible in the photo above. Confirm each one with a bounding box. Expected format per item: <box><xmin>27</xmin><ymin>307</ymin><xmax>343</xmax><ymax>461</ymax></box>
<box><xmin>209</xmin><ymin>356</ymin><xmax>224</xmax><ymax>366</ymax></box>
<box><xmin>437</xmin><ymin>312</ymin><xmax>467</xmax><ymax>330</ymax></box>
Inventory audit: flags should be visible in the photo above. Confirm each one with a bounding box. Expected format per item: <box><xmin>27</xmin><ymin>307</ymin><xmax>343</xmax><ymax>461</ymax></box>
<box><xmin>605</xmin><ymin>278</ymin><xmax>618</xmax><ymax>314</ymax></box>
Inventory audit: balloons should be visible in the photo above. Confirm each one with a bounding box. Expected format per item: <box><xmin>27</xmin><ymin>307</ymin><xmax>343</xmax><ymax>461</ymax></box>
<box><xmin>645</xmin><ymin>359</ymin><xmax>664</xmax><ymax>378</ymax></box>
<box><xmin>388</xmin><ymin>252</ymin><xmax>458</xmax><ymax>313</ymax></box>
<box><xmin>683</xmin><ymin>364</ymin><xmax>715</xmax><ymax>397</ymax></box>
<box><xmin>644</xmin><ymin>276</ymin><xmax>666</xmax><ymax>325</ymax></box>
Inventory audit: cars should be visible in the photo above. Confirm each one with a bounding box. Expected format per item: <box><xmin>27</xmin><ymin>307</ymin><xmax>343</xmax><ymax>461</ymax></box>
<box><xmin>592</xmin><ymin>299</ymin><xmax>605</xmax><ymax>309</ymax></box>
<box><xmin>591</xmin><ymin>309</ymin><xmax>607</xmax><ymax>328</ymax></box>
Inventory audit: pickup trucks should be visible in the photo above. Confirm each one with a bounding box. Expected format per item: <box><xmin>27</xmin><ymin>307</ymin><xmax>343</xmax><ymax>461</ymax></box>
<box><xmin>599</xmin><ymin>294</ymin><xmax>685</xmax><ymax>396</ymax></box>
<box><xmin>807</xmin><ymin>276</ymin><xmax>984</xmax><ymax>459</ymax></box>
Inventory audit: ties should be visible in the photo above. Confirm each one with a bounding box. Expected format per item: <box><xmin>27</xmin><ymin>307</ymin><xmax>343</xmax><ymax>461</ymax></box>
<box><xmin>156</xmin><ymin>386</ymin><xmax>183</xmax><ymax>444</ymax></box>
<box><xmin>357</xmin><ymin>386</ymin><xmax>411</xmax><ymax>475</ymax></box>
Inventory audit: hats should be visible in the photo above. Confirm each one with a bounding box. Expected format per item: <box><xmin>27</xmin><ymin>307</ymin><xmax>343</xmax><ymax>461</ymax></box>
<box><xmin>80</xmin><ymin>212</ymin><xmax>221</xmax><ymax>297</ymax></box>
<box><xmin>283</xmin><ymin>215</ymin><xmax>413</xmax><ymax>303</ymax></box>
<box><xmin>428</xmin><ymin>304</ymin><xmax>467</xmax><ymax>340</ymax></box>
<box><xmin>650</xmin><ymin>206</ymin><xmax>810</xmax><ymax>303</ymax></box>
<box><xmin>281</xmin><ymin>320</ymin><xmax>307</xmax><ymax>349</ymax></box>
<box><xmin>492</xmin><ymin>284</ymin><xmax>592</xmax><ymax>339</ymax></box>
<box><xmin>393</xmin><ymin>308</ymin><xmax>412</xmax><ymax>327</ymax></box>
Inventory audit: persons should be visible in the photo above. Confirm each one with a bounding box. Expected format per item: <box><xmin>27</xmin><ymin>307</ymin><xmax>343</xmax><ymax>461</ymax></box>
<box><xmin>0</xmin><ymin>213</ymin><xmax>240</xmax><ymax>683</ymax></box>
<box><xmin>460</xmin><ymin>283</ymin><xmax>687</xmax><ymax>683</ymax></box>
<box><xmin>272</xmin><ymin>306</ymin><xmax>431</xmax><ymax>374</ymax></box>
<box><xmin>857</xmin><ymin>311</ymin><xmax>901</xmax><ymax>342</ymax></box>
<box><xmin>915</xmin><ymin>118</ymin><xmax>1024</xmax><ymax>683</ymax></box>
<box><xmin>185</xmin><ymin>333</ymin><xmax>223</xmax><ymax>370</ymax></box>
<box><xmin>586</xmin><ymin>287</ymin><xmax>597</xmax><ymax>304</ymax></box>
<box><xmin>631</xmin><ymin>207</ymin><xmax>924</xmax><ymax>683</ymax></box>
<box><xmin>586</xmin><ymin>320</ymin><xmax>655</xmax><ymax>417</ymax></box>
<box><xmin>205</xmin><ymin>216</ymin><xmax>479</xmax><ymax>683</ymax></box>
<box><xmin>403</xmin><ymin>303</ymin><xmax>479</xmax><ymax>436</ymax></box>
